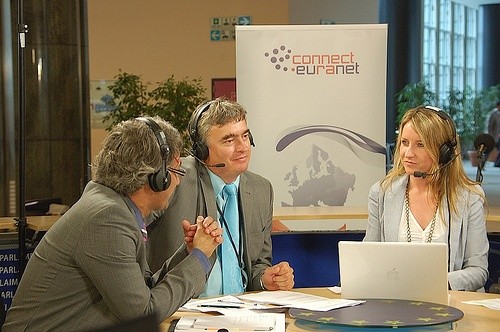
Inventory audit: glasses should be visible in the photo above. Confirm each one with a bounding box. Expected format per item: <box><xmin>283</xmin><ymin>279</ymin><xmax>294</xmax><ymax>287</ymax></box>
<box><xmin>168</xmin><ymin>166</ymin><xmax>186</xmax><ymax>178</ymax></box>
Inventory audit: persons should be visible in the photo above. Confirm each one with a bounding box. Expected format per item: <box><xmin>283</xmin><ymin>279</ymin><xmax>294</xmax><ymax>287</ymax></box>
<box><xmin>487</xmin><ymin>102</ymin><xmax>500</xmax><ymax>167</ymax></box>
<box><xmin>361</xmin><ymin>106</ymin><xmax>489</xmax><ymax>294</ymax></box>
<box><xmin>1</xmin><ymin>114</ymin><xmax>224</xmax><ymax>332</ymax></box>
<box><xmin>145</xmin><ymin>95</ymin><xmax>295</xmax><ymax>299</ymax></box>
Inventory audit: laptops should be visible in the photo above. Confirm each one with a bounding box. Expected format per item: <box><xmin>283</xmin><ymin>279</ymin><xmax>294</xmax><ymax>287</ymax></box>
<box><xmin>338</xmin><ymin>241</ymin><xmax>451</xmax><ymax>307</ymax></box>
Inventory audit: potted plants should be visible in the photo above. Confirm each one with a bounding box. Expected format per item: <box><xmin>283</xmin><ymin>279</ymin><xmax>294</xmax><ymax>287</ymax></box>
<box><xmin>453</xmin><ymin>88</ymin><xmax>500</xmax><ymax>164</ymax></box>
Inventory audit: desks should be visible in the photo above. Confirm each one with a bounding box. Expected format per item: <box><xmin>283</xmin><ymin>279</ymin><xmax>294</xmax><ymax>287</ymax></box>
<box><xmin>154</xmin><ymin>289</ymin><xmax>500</xmax><ymax>332</ymax></box>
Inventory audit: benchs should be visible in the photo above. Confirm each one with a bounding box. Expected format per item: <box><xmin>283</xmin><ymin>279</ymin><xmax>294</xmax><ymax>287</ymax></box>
<box><xmin>272</xmin><ymin>229</ymin><xmax>500</xmax><ymax>290</ymax></box>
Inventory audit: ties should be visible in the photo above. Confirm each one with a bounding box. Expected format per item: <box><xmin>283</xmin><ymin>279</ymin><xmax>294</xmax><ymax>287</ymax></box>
<box><xmin>220</xmin><ymin>183</ymin><xmax>245</xmax><ymax>295</ymax></box>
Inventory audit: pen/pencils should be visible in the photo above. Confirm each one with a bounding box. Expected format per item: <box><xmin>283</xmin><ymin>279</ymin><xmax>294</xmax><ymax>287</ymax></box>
<box><xmin>198</xmin><ymin>304</ymin><xmax>244</xmax><ymax>308</ymax></box>
<box><xmin>218</xmin><ymin>300</ymin><xmax>258</xmax><ymax>305</ymax></box>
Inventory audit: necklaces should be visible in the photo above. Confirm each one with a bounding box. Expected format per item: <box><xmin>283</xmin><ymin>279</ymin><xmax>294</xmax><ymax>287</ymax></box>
<box><xmin>405</xmin><ymin>183</ymin><xmax>442</xmax><ymax>243</ymax></box>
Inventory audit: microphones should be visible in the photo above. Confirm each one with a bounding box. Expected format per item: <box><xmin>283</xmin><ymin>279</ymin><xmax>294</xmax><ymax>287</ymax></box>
<box><xmin>184</xmin><ymin>148</ymin><xmax>225</xmax><ymax>167</ymax></box>
<box><xmin>413</xmin><ymin>151</ymin><xmax>463</xmax><ymax>177</ymax></box>
<box><xmin>473</xmin><ymin>134</ymin><xmax>495</xmax><ymax>158</ymax></box>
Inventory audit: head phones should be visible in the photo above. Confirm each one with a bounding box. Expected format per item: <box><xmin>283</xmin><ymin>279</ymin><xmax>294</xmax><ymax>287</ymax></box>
<box><xmin>418</xmin><ymin>105</ymin><xmax>458</xmax><ymax>165</ymax></box>
<box><xmin>134</xmin><ymin>117</ymin><xmax>172</xmax><ymax>192</ymax></box>
<box><xmin>189</xmin><ymin>102</ymin><xmax>255</xmax><ymax>162</ymax></box>
